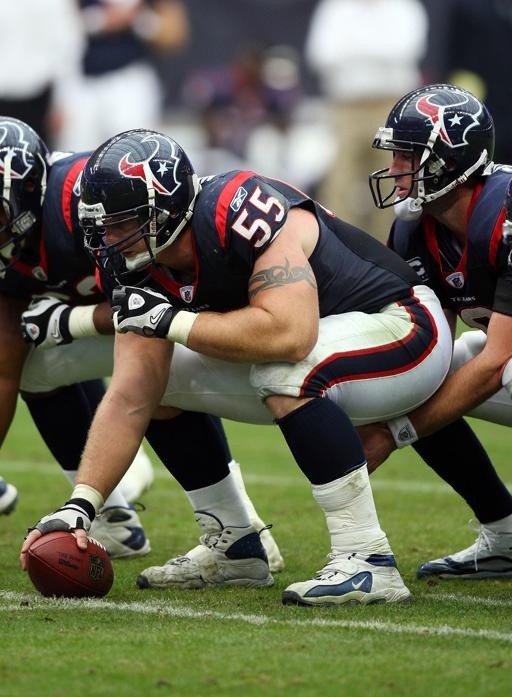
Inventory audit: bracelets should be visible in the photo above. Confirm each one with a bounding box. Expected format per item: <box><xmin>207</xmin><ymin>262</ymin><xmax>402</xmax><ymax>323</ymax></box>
<box><xmin>388</xmin><ymin>414</ymin><xmax>418</xmax><ymax>450</ymax></box>
<box><xmin>166</xmin><ymin>308</ymin><xmax>199</xmax><ymax>349</ymax></box>
<box><xmin>70</xmin><ymin>302</ymin><xmax>103</xmax><ymax>340</ymax></box>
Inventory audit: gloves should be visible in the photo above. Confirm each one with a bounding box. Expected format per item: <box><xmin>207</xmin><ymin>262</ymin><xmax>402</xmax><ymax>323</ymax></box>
<box><xmin>111</xmin><ymin>285</ymin><xmax>178</xmax><ymax>340</ymax></box>
<box><xmin>20</xmin><ymin>294</ymin><xmax>73</xmax><ymax>349</ymax></box>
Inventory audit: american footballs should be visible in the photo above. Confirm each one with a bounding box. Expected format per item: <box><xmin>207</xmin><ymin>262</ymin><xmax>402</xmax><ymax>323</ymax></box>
<box><xmin>27</xmin><ymin>530</ymin><xmax>113</xmax><ymax>598</ymax></box>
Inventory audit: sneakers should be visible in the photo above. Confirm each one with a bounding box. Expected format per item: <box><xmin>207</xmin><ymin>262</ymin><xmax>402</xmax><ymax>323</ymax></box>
<box><xmin>0</xmin><ymin>475</ymin><xmax>19</xmax><ymax>516</ymax></box>
<box><xmin>417</xmin><ymin>527</ymin><xmax>512</xmax><ymax>580</ymax></box>
<box><xmin>136</xmin><ymin>510</ymin><xmax>284</xmax><ymax>590</ymax></box>
<box><xmin>88</xmin><ymin>447</ymin><xmax>153</xmax><ymax>558</ymax></box>
<box><xmin>282</xmin><ymin>553</ymin><xmax>411</xmax><ymax>606</ymax></box>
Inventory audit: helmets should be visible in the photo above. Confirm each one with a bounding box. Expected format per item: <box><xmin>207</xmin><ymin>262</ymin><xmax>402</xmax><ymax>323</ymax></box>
<box><xmin>77</xmin><ymin>128</ymin><xmax>201</xmax><ymax>289</ymax></box>
<box><xmin>368</xmin><ymin>82</ymin><xmax>496</xmax><ymax>209</ymax></box>
<box><xmin>0</xmin><ymin>116</ymin><xmax>51</xmax><ymax>271</ymax></box>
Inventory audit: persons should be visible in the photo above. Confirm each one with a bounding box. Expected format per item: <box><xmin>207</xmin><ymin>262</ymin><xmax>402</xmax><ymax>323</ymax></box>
<box><xmin>355</xmin><ymin>83</ymin><xmax>511</xmax><ymax>584</ymax></box>
<box><xmin>0</xmin><ymin>0</ymin><xmax>505</xmax><ymax>249</ymax></box>
<box><xmin>0</xmin><ymin>114</ymin><xmax>285</xmax><ymax>576</ymax></box>
<box><xmin>19</xmin><ymin>126</ymin><xmax>455</xmax><ymax>607</ymax></box>
